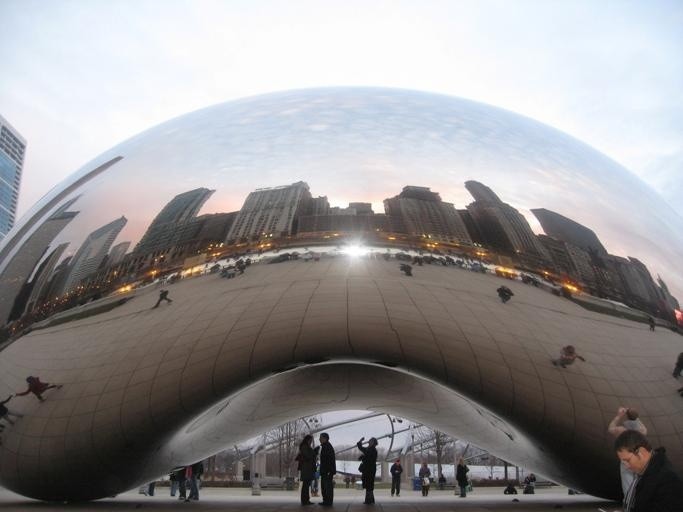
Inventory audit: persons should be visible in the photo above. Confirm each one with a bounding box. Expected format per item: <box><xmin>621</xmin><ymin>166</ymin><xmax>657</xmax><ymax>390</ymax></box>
<box><xmin>551</xmin><ymin>345</ymin><xmax>585</xmax><ymax>367</ymax></box>
<box><xmin>499</xmin><ymin>287</ymin><xmax>514</xmax><ymax>305</ymax></box>
<box><xmin>530</xmin><ymin>474</ymin><xmax>536</xmax><ymax>482</ymax></box>
<box><xmin>672</xmin><ymin>351</ymin><xmax>683</xmax><ymax>398</ymax></box>
<box><xmin>455</xmin><ymin>457</ymin><xmax>469</xmax><ymax>497</ymax></box>
<box><xmin>251</xmin><ymin>472</ymin><xmax>261</xmax><ymax>496</ymax></box>
<box><xmin>496</xmin><ymin>284</ymin><xmax>505</xmax><ymax>297</ymax></box>
<box><xmin>150</xmin><ymin>289</ymin><xmax>173</xmax><ymax>308</ymax></box>
<box><xmin>615</xmin><ymin>429</ymin><xmax>682</xmax><ymax>511</ymax></box>
<box><xmin>524</xmin><ymin>471</ymin><xmax>533</xmax><ymax>492</ymax></box>
<box><xmin>317</xmin><ymin>433</ymin><xmax>336</xmax><ymax>506</ymax></box>
<box><xmin>356</xmin><ymin>436</ymin><xmax>378</xmax><ymax>503</ymax></box>
<box><xmin>648</xmin><ymin>316</ymin><xmax>655</xmax><ymax>332</ymax></box>
<box><xmin>389</xmin><ymin>459</ymin><xmax>403</xmax><ymax>497</ymax></box>
<box><xmin>310</xmin><ymin>458</ymin><xmax>319</xmax><ymax>498</ymax></box>
<box><xmin>141</xmin><ymin>461</ymin><xmax>203</xmax><ymax>502</ymax></box>
<box><xmin>418</xmin><ymin>462</ymin><xmax>431</xmax><ymax>496</ymax></box>
<box><xmin>210</xmin><ymin>259</ymin><xmax>251</xmax><ymax>281</ymax></box>
<box><xmin>0</xmin><ymin>395</ymin><xmax>24</xmax><ymax>427</ymax></box>
<box><xmin>503</xmin><ymin>480</ymin><xmax>517</xmax><ymax>494</ymax></box>
<box><xmin>299</xmin><ymin>435</ymin><xmax>319</xmax><ymax>506</ymax></box>
<box><xmin>399</xmin><ymin>263</ymin><xmax>413</xmax><ymax>275</ymax></box>
<box><xmin>11</xmin><ymin>376</ymin><xmax>63</xmax><ymax>402</ymax></box>
<box><xmin>608</xmin><ymin>406</ymin><xmax>647</xmax><ymax>509</ymax></box>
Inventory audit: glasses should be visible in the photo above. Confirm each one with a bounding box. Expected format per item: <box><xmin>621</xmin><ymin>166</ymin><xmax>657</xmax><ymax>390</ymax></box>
<box><xmin>620</xmin><ymin>454</ymin><xmax>636</xmax><ymax>466</ymax></box>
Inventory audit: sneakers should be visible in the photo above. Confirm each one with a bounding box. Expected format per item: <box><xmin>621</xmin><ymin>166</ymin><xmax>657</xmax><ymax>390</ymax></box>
<box><xmin>179</xmin><ymin>495</ymin><xmax>199</xmax><ymax>502</ymax></box>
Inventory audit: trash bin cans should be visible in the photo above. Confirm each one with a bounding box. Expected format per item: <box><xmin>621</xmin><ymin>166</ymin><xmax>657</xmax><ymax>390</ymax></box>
<box><xmin>286</xmin><ymin>477</ymin><xmax>295</xmax><ymax>490</ymax></box>
<box><xmin>414</xmin><ymin>477</ymin><xmax>422</xmax><ymax>491</ymax></box>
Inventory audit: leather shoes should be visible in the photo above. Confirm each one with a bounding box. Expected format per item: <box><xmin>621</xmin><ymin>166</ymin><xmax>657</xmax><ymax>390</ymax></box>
<box><xmin>318</xmin><ymin>501</ymin><xmax>332</xmax><ymax>505</ymax></box>
<box><xmin>302</xmin><ymin>500</ymin><xmax>314</xmax><ymax>505</ymax></box>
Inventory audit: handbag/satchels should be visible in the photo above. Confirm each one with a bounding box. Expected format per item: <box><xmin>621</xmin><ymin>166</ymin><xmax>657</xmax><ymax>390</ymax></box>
<box><xmin>295</xmin><ymin>451</ymin><xmax>310</xmax><ymax>461</ymax></box>
<box><xmin>422</xmin><ymin>478</ymin><xmax>430</xmax><ymax>486</ymax></box>
